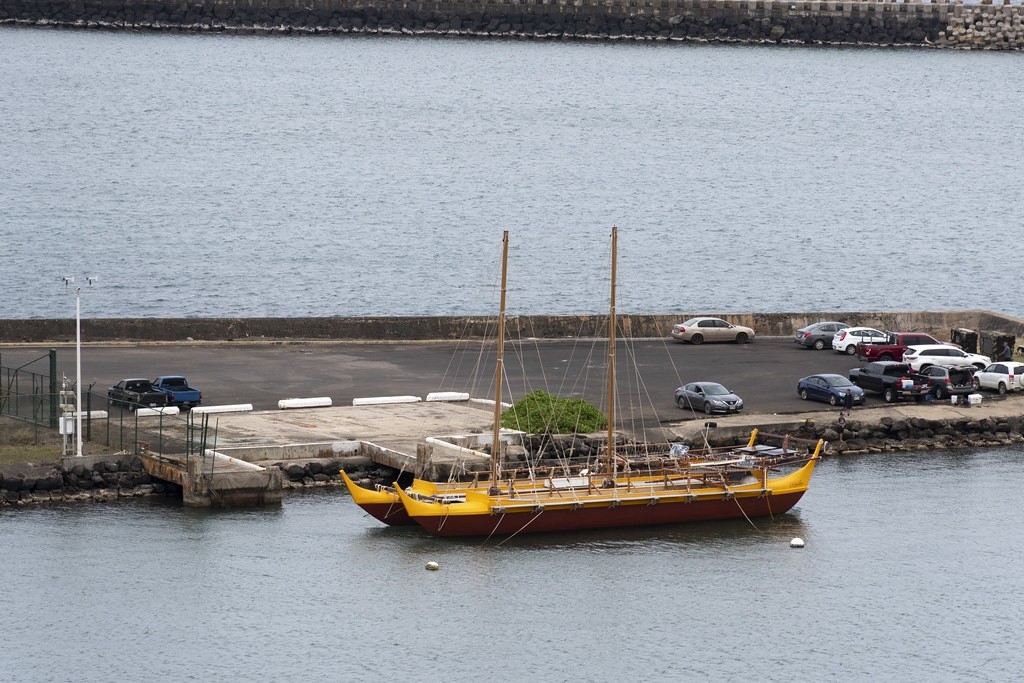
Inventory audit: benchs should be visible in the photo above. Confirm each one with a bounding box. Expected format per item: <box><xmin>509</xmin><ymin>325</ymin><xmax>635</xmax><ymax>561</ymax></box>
<box><xmin>543</xmin><ymin>477</ymin><xmax>592</xmax><ymax>489</ymax></box>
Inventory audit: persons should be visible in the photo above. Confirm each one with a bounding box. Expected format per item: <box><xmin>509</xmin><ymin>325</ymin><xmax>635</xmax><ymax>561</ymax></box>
<box><xmin>999</xmin><ymin>342</ymin><xmax>1011</xmax><ymax>361</ymax></box>
<box><xmin>838</xmin><ymin>411</ymin><xmax>845</xmax><ymax>442</ymax></box>
<box><xmin>843</xmin><ymin>389</ymin><xmax>853</xmax><ymax>416</ymax></box>
<box><xmin>907</xmin><ymin>363</ymin><xmax>915</xmax><ymax>374</ymax></box>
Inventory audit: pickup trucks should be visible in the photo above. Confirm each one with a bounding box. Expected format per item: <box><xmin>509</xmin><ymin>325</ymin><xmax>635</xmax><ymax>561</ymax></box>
<box><xmin>856</xmin><ymin>332</ymin><xmax>962</xmax><ymax>363</ymax></box>
<box><xmin>106</xmin><ymin>378</ymin><xmax>169</xmax><ymax>411</ymax></box>
<box><xmin>150</xmin><ymin>375</ymin><xmax>202</xmax><ymax>408</ymax></box>
<box><xmin>848</xmin><ymin>361</ymin><xmax>932</xmax><ymax>402</ymax></box>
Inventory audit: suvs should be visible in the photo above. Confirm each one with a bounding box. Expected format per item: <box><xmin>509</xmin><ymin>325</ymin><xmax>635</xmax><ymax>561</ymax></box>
<box><xmin>902</xmin><ymin>345</ymin><xmax>993</xmax><ymax>374</ymax></box>
<box><xmin>972</xmin><ymin>361</ymin><xmax>1024</xmax><ymax>395</ymax></box>
<box><xmin>918</xmin><ymin>364</ymin><xmax>980</xmax><ymax>399</ymax></box>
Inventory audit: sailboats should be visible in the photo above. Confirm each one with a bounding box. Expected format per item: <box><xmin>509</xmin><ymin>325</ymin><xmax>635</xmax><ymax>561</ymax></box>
<box><xmin>337</xmin><ymin>226</ymin><xmax>827</xmax><ymax>541</ymax></box>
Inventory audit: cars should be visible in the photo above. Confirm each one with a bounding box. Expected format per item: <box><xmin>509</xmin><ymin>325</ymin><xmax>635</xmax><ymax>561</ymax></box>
<box><xmin>797</xmin><ymin>374</ymin><xmax>867</xmax><ymax>406</ymax></box>
<box><xmin>671</xmin><ymin>316</ymin><xmax>756</xmax><ymax>345</ymax></box>
<box><xmin>673</xmin><ymin>381</ymin><xmax>744</xmax><ymax>416</ymax></box>
<box><xmin>795</xmin><ymin>321</ymin><xmax>852</xmax><ymax>350</ymax></box>
<box><xmin>832</xmin><ymin>326</ymin><xmax>890</xmax><ymax>356</ymax></box>
